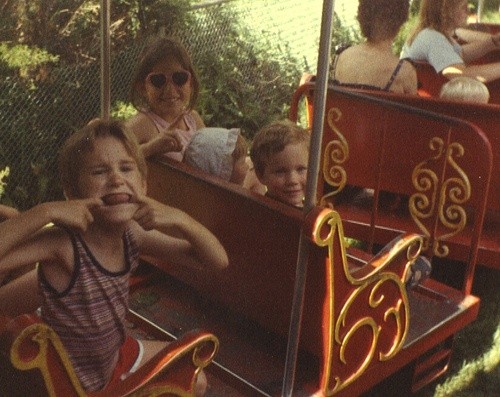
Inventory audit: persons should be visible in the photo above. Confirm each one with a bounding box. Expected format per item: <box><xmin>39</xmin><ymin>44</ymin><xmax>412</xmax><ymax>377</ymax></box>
<box><xmin>122</xmin><ymin>37</ymin><xmax>267</xmax><ymax>195</ymax></box>
<box><xmin>332</xmin><ymin>0</ymin><xmax>418</xmax><ymax>97</ymax></box>
<box><xmin>1</xmin><ymin>119</ymin><xmax>229</xmax><ymax>397</ymax></box>
<box><xmin>401</xmin><ymin>0</ymin><xmax>500</xmax><ymax>82</ymax></box>
<box><xmin>185</xmin><ymin>125</ymin><xmax>249</xmax><ymax>188</ymax></box>
<box><xmin>438</xmin><ymin>75</ymin><xmax>490</xmax><ymax>107</ymax></box>
<box><xmin>252</xmin><ymin>119</ymin><xmax>312</xmax><ymax>207</ymax></box>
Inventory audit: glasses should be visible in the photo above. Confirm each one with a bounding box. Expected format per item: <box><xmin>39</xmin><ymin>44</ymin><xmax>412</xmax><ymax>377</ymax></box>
<box><xmin>145</xmin><ymin>68</ymin><xmax>191</xmax><ymax>89</ymax></box>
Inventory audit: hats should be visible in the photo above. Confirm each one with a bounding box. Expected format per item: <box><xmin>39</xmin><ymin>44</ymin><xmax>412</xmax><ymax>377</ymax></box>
<box><xmin>181</xmin><ymin>126</ymin><xmax>241</xmax><ymax>184</ymax></box>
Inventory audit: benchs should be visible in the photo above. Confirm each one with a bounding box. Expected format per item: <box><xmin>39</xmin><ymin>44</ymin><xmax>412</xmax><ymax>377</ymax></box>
<box><xmin>0</xmin><ymin>23</ymin><xmax>500</xmax><ymax>397</ymax></box>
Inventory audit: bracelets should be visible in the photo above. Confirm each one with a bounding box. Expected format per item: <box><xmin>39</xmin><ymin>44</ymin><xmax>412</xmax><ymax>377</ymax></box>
<box><xmin>491</xmin><ymin>36</ymin><xmax>499</xmax><ymax>47</ymax></box>
